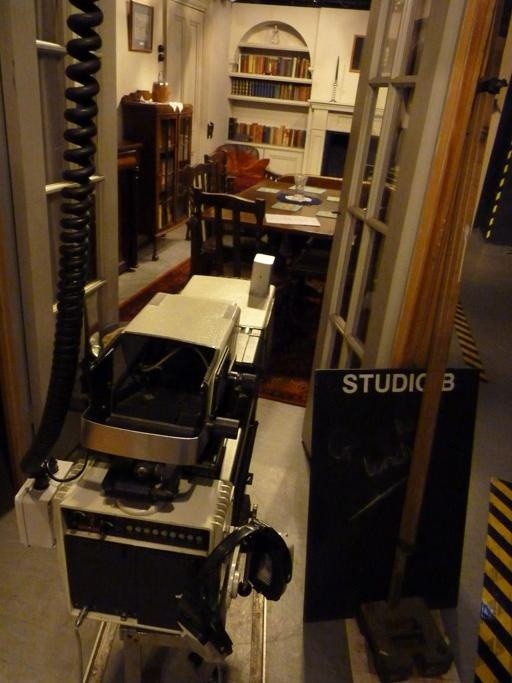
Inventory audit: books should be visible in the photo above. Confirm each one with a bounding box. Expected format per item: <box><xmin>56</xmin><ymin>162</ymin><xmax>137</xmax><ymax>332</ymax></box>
<box><xmin>226</xmin><ymin>49</ymin><xmax>312</xmax><ymax>148</ymax></box>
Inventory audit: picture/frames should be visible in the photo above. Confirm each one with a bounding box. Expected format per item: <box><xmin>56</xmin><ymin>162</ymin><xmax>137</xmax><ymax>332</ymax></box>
<box><xmin>128</xmin><ymin>0</ymin><xmax>157</xmax><ymax>57</ymax></box>
<box><xmin>350</xmin><ymin>30</ymin><xmax>398</xmax><ymax>75</ymax></box>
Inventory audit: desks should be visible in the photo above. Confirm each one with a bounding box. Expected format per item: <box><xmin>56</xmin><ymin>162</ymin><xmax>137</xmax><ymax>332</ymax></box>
<box><xmin>197</xmin><ymin>179</ymin><xmax>387</xmax><ymax>286</ymax></box>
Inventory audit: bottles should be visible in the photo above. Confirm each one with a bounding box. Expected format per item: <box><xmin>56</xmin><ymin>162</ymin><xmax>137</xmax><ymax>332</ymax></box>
<box><xmin>151</xmin><ymin>82</ymin><xmax>169</xmax><ymax>102</ymax></box>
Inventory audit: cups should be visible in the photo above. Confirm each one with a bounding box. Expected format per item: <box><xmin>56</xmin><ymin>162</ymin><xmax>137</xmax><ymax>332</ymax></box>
<box><xmin>294</xmin><ymin>174</ymin><xmax>308</xmax><ymax>200</ymax></box>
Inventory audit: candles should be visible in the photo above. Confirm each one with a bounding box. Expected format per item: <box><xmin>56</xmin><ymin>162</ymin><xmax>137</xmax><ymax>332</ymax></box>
<box><xmin>332</xmin><ymin>55</ymin><xmax>341</xmax><ymax>83</ymax></box>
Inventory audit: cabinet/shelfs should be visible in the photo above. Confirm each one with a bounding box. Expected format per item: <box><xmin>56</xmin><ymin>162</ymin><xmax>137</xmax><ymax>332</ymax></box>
<box><xmin>229</xmin><ymin>38</ymin><xmax>313</xmax><ymax>112</ymax></box>
<box><xmin>120</xmin><ymin>95</ymin><xmax>194</xmax><ymax>263</ymax></box>
<box><xmin>115</xmin><ymin>135</ymin><xmax>142</xmax><ymax>281</ymax></box>
<box><xmin>224</xmin><ymin>136</ymin><xmax>308</xmax><ymax>178</ymax></box>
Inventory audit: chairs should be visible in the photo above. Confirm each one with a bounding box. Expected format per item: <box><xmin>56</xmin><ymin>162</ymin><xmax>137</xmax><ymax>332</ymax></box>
<box><xmin>188</xmin><ymin>181</ymin><xmax>288</xmax><ymax>311</ymax></box>
<box><xmin>177</xmin><ymin>148</ymin><xmax>259</xmax><ymax>267</ymax></box>
<box><xmin>302</xmin><ymin>173</ymin><xmax>376</xmax><ymax>193</ymax></box>
<box><xmin>289</xmin><ymin>175</ymin><xmax>398</xmax><ymax>343</ymax></box>
<box><xmin>204</xmin><ymin>137</ymin><xmax>294</xmax><ymax>195</ymax></box>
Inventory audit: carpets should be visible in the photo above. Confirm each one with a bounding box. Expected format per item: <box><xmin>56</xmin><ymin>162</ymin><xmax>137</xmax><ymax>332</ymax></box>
<box><xmin>86</xmin><ymin>250</ymin><xmax>374</xmax><ymax>409</ymax></box>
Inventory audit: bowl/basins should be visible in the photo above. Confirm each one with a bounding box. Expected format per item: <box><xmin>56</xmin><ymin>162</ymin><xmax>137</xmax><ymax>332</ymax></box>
<box><xmin>137</xmin><ymin>91</ymin><xmax>151</xmax><ymax>100</ymax></box>
